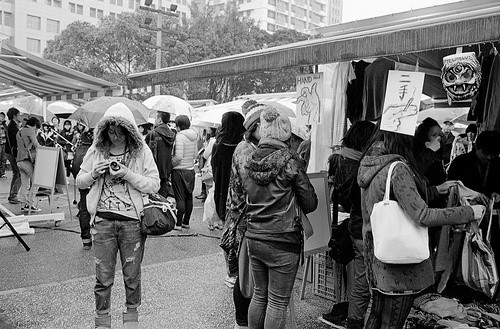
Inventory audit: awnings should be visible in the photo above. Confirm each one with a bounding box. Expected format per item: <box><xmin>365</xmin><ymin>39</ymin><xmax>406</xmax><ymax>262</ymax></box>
<box><xmin>0</xmin><ymin>41</ymin><xmax>124</xmax><ymax>103</ymax></box>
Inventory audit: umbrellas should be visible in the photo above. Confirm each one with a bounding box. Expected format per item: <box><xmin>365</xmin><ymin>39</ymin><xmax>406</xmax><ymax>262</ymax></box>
<box><xmin>193</xmin><ymin>109</ymin><xmax>224</xmax><ymax>129</ymax></box>
<box><xmin>68</xmin><ymin>95</ymin><xmax>151</xmax><ymax>129</ymax></box>
<box><xmin>142</xmin><ymin>95</ymin><xmax>200</xmax><ymax>117</ymax></box>
<box><xmin>258</xmin><ymin>98</ymin><xmax>309</xmax><ymax>140</ymax></box>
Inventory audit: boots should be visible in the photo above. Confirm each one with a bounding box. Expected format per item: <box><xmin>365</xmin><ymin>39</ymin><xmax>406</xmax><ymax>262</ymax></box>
<box><xmin>95</xmin><ymin>312</ymin><xmax>111</xmax><ymax>329</ymax></box>
<box><xmin>195</xmin><ymin>183</ymin><xmax>206</xmax><ymax>199</ymax></box>
<box><xmin>123</xmin><ymin>311</ymin><xmax>139</xmax><ymax>329</ymax></box>
<box><xmin>66</xmin><ymin>177</ymin><xmax>70</xmax><ymax>184</ymax></box>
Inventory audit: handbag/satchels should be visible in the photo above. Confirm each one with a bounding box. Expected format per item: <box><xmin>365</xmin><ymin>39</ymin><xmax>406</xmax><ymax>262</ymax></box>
<box><xmin>139</xmin><ymin>191</ymin><xmax>177</xmax><ymax>236</ymax></box>
<box><xmin>461</xmin><ymin>224</ymin><xmax>499</xmax><ymax>298</ymax></box>
<box><xmin>370</xmin><ymin>161</ymin><xmax>430</xmax><ymax>263</ymax></box>
<box><xmin>327</xmin><ymin>217</ymin><xmax>355</xmax><ymax>265</ymax></box>
<box><xmin>203</xmin><ymin>182</ymin><xmax>221</xmax><ymax>223</ymax></box>
<box><xmin>238</xmin><ymin>235</ymin><xmax>255</xmax><ymax>298</ymax></box>
<box><xmin>27</xmin><ymin>148</ymin><xmax>36</xmax><ymax>163</ymax></box>
<box><xmin>67</xmin><ymin>152</ymin><xmax>74</xmax><ymax>160</ymax></box>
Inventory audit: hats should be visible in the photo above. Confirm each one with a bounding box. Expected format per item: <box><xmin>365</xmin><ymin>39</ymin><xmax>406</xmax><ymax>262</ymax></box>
<box><xmin>443</xmin><ymin>121</ymin><xmax>453</xmax><ymax>125</ymax></box>
<box><xmin>260</xmin><ymin>106</ymin><xmax>291</xmax><ymax>141</ymax></box>
<box><xmin>241</xmin><ymin>100</ymin><xmax>268</xmax><ymax>129</ymax></box>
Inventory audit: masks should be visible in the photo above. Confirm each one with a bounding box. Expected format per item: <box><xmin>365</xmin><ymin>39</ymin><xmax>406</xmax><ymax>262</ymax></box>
<box><xmin>78</xmin><ymin>126</ymin><xmax>83</xmax><ymax>130</ymax></box>
<box><xmin>64</xmin><ymin>125</ymin><xmax>71</xmax><ymax>130</ymax></box>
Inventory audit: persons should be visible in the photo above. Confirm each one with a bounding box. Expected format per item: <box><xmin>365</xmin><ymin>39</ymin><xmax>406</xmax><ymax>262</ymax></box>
<box><xmin>36</xmin><ymin>116</ymin><xmax>95</xmax><ymax>250</ymax></box>
<box><xmin>0</xmin><ymin>112</ymin><xmax>8</xmax><ymax>178</ymax></box>
<box><xmin>325</xmin><ymin>117</ymin><xmax>500</xmax><ymax>329</ymax></box>
<box><xmin>5</xmin><ymin>107</ymin><xmax>24</xmax><ymax>204</ymax></box>
<box><xmin>210</xmin><ymin>100</ymin><xmax>319</xmax><ymax>329</ymax></box>
<box><xmin>16</xmin><ymin>117</ymin><xmax>42</xmax><ymax>212</ymax></box>
<box><xmin>76</xmin><ymin>102</ymin><xmax>161</xmax><ymax>329</ymax></box>
<box><xmin>137</xmin><ymin>110</ymin><xmax>218</xmax><ymax>232</ymax></box>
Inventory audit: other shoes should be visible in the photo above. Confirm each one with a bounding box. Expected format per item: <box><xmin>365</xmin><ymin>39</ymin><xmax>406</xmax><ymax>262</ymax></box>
<box><xmin>225</xmin><ymin>274</ymin><xmax>238</xmax><ymax>287</ymax></box>
<box><xmin>182</xmin><ymin>224</ymin><xmax>190</xmax><ymax>228</ymax></box>
<box><xmin>84</xmin><ymin>245</ymin><xmax>92</xmax><ymax>249</ymax></box>
<box><xmin>174</xmin><ymin>225</ymin><xmax>182</xmax><ymax>231</ymax></box>
<box><xmin>8</xmin><ymin>196</ymin><xmax>23</xmax><ymax>204</ymax></box>
<box><xmin>208</xmin><ymin>223</ymin><xmax>223</xmax><ymax>231</ymax></box>
<box><xmin>21</xmin><ymin>204</ymin><xmax>42</xmax><ymax>212</ymax></box>
